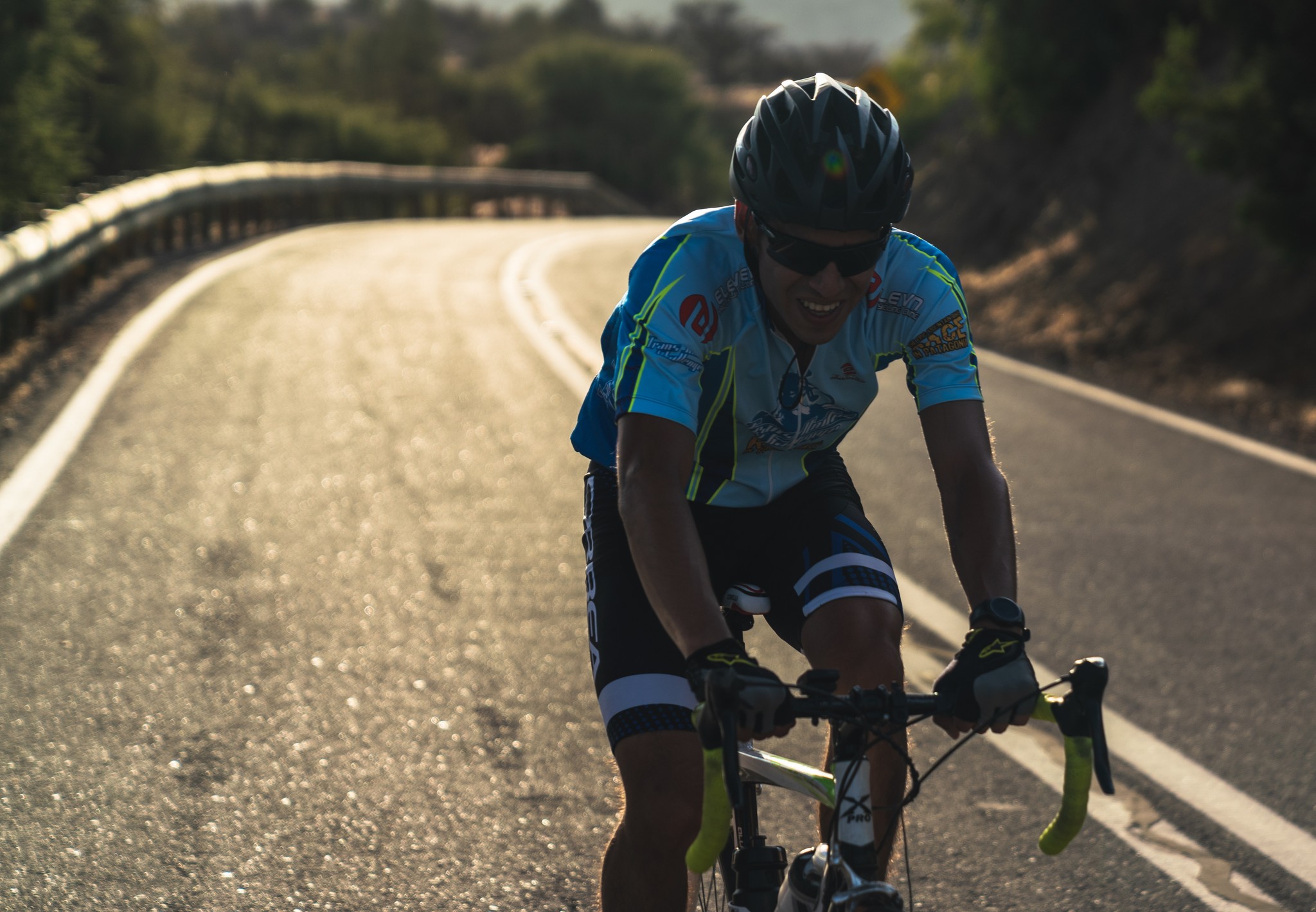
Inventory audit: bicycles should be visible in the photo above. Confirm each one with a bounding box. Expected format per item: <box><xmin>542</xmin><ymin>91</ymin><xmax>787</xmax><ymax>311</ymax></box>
<box><xmin>684</xmin><ymin>580</ymin><xmax>1114</xmax><ymax>912</ymax></box>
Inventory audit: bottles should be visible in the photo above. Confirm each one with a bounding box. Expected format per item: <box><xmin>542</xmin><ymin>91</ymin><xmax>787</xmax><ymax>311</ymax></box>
<box><xmin>769</xmin><ymin>841</ymin><xmax>829</xmax><ymax>911</ymax></box>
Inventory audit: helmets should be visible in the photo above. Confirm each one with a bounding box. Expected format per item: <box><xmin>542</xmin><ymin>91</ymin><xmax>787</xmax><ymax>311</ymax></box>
<box><xmin>729</xmin><ymin>72</ymin><xmax>916</xmax><ymax>231</ymax></box>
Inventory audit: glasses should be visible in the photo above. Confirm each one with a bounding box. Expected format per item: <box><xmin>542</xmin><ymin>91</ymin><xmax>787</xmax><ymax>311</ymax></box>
<box><xmin>752</xmin><ymin>210</ymin><xmax>892</xmax><ymax>277</ymax></box>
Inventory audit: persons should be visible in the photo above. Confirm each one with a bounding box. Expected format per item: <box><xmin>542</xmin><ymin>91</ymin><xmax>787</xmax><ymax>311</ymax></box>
<box><xmin>571</xmin><ymin>68</ymin><xmax>1043</xmax><ymax>912</ymax></box>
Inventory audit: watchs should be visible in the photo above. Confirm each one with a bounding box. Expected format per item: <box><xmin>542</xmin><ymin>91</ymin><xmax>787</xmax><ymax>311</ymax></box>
<box><xmin>968</xmin><ymin>596</ymin><xmax>1025</xmax><ymax>631</ymax></box>
